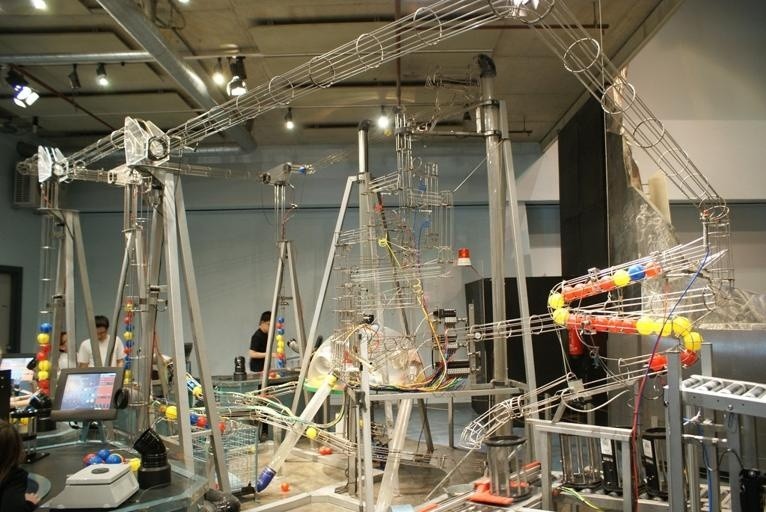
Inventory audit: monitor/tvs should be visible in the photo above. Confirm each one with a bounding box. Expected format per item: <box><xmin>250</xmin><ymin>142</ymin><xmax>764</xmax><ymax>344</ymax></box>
<box><xmin>0</xmin><ymin>354</ymin><xmax>38</xmax><ymax>383</ymax></box>
<box><xmin>50</xmin><ymin>367</ymin><xmax>125</xmax><ymax>422</ymax></box>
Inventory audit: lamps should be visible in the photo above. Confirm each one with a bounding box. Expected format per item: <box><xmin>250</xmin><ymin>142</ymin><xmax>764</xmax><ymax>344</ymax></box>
<box><xmin>225</xmin><ymin>57</ymin><xmax>249</xmax><ymax>97</ymax></box>
<box><xmin>66</xmin><ymin>64</ymin><xmax>82</xmax><ymax>89</ymax></box>
<box><xmin>378</xmin><ymin>105</ymin><xmax>389</xmax><ymax>128</ymax></box>
<box><xmin>5</xmin><ymin>65</ymin><xmax>39</xmax><ymax>107</ymax></box>
<box><xmin>212</xmin><ymin>56</ymin><xmax>224</xmax><ymax>84</ymax></box>
<box><xmin>284</xmin><ymin>108</ymin><xmax>294</xmax><ymax>129</ymax></box>
<box><xmin>95</xmin><ymin>64</ymin><xmax>108</xmax><ymax>87</ymax></box>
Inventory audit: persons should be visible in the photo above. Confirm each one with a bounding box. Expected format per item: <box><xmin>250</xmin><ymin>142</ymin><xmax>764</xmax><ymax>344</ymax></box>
<box><xmin>78</xmin><ymin>314</ymin><xmax>128</xmax><ymax>367</ymax></box>
<box><xmin>247</xmin><ymin>311</ymin><xmax>276</xmax><ymax>372</ymax></box>
<box><xmin>58</xmin><ymin>331</ymin><xmax>79</xmax><ymax>370</ymax></box>
<box><xmin>0</xmin><ymin>419</ymin><xmax>40</xmax><ymax>512</ymax></box>
